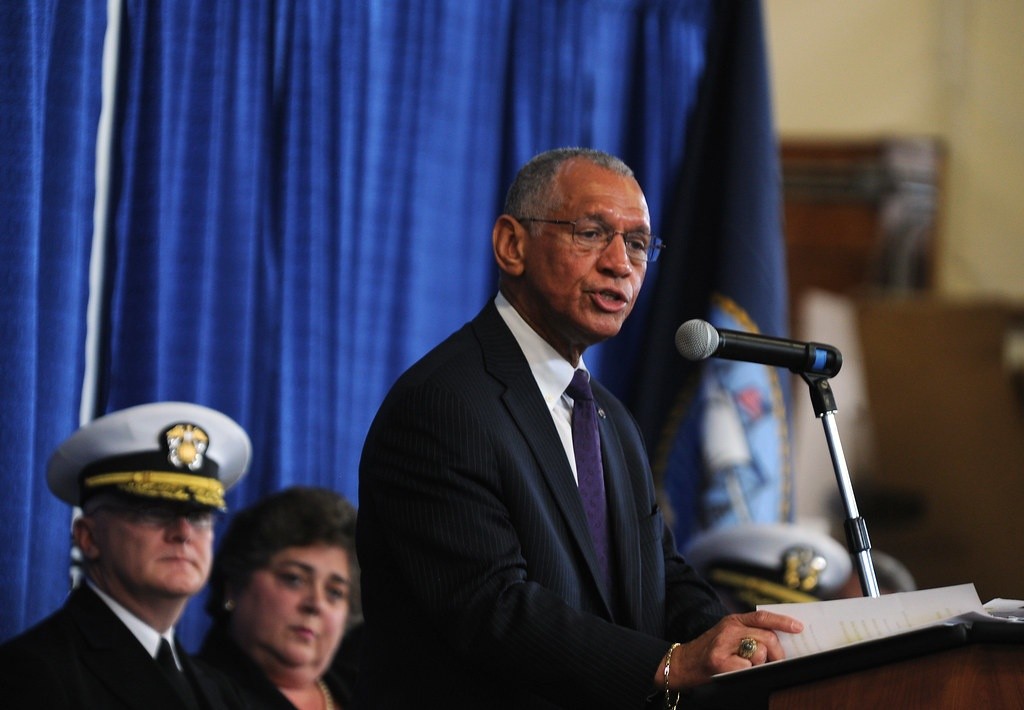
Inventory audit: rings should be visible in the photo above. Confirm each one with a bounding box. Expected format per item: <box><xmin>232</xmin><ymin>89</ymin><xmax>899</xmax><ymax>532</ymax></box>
<box><xmin>736</xmin><ymin>636</ymin><xmax>758</xmax><ymax>658</ymax></box>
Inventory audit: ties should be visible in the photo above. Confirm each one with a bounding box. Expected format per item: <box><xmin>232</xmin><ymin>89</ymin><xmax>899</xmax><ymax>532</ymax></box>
<box><xmin>156</xmin><ymin>637</ymin><xmax>200</xmax><ymax>709</ymax></box>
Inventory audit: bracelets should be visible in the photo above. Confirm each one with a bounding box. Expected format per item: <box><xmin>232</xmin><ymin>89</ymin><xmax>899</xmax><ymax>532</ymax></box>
<box><xmin>662</xmin><ymin>642</ymin><xmax>682</xmax><ymax>709</ymax></box>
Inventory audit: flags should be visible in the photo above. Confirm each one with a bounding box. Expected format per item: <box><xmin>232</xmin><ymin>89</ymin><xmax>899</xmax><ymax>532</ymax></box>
<box><xmin>617</xmin><ymin>2</ymin><xmax>792</xmax><ymax>539</ymax></box>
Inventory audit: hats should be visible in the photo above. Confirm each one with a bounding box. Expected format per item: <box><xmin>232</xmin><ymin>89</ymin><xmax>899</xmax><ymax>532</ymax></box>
<box><xmin>679</xmin><ymin>522</ymin><xmax>852</xmax><ymax>611</ymax></box>
<box><xmin>46</xmin><ymin>402</ymin><xmax>253</xmax><ymax>519</ymax></box>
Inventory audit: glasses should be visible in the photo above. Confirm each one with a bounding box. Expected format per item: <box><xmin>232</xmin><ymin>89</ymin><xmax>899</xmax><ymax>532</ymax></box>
<box><xmin>518</xmin><ymin>216</ymin><xmax>668</xmax><ymax>263</ymax></box>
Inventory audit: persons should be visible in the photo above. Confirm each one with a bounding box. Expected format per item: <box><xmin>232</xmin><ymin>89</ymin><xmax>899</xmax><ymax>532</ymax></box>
<box><xmin>356</xmin><ymin>140</ymin><xmax>805</xmax><ymax>710</ymax></box>
<box><xmin>0</xmin><ymin>402</ymin><xmax>257</xmax><ymax>710</ymax></box>
<box><xmin>139</xmin><ymin>480</ymin><xmax>371</xmax><ymax>710</ymax></box>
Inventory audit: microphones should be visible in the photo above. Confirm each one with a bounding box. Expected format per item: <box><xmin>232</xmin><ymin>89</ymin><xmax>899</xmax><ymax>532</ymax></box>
<box><xmin>674</xmin><ymin>319</ymin><xmax>843</xmax><ymax>380</ymax></box>
